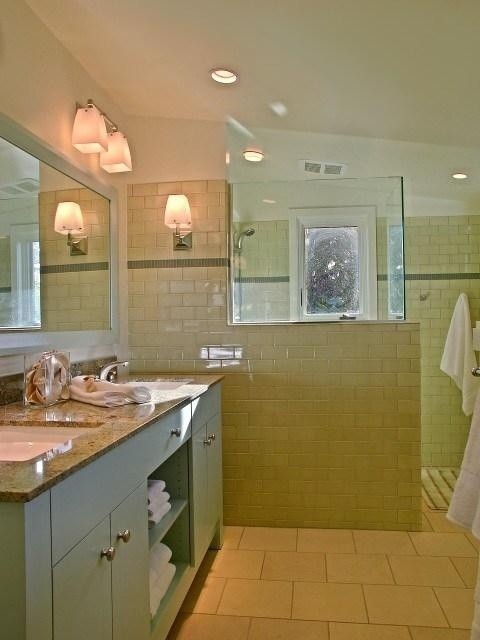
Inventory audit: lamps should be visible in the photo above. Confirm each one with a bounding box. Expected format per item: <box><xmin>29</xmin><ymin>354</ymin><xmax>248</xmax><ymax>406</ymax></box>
<box><xmin>164</xmin><ymin>193</ymin><xmax>192</xmax><ymax>249</ymax></box>
<box><xmin>54</xmin><ymin>201</ymin><xmax>88</xmax><ymax>255</ymax></box>
<box><xmin>70</xmin><ymin>98</ymin><xmax>132</xmax><ymax>175</ymax></box>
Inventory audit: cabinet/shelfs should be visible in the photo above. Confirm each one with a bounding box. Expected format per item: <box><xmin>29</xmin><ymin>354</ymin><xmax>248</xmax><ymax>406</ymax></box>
<box><xmin>51</xmin><ymin>479</ymin><xmax>150</xmax><ymax>640</ymax></box>
<box><xmin>191</xmin><ymin>414</ymin><xmax>222</xmax><ymax>568</ymax></box>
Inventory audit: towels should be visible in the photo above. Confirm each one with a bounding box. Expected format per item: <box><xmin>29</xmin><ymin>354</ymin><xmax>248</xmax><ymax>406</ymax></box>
<box><xmin>444</xmin><ymin>384</ymin><xmax>480</xmax><ymax>540</ymax></box>
<box><xmin>149</xmin><ymin>543</ymin><xmax>176</xmax><ymax>619</ymax></box>
<box><xmin>146</xmin><ymin>479</ymin><xmax>171</xmax><ymax>529</ymax></box>
<box><xmin>440</xmin><ymin>293</ymin><xmax>478</xmax><ymax>417</ymax></box>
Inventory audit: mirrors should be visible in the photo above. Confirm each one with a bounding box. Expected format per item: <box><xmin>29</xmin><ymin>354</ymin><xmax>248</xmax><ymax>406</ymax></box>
<box><xmin>224</xmin><ymin>176</ymin><xmax>405</xmax><ymax>329</ymax></box>
<box><xmin>0</xmin><ymin>135</ymin><xmax>111</xmax><ymax>333</ymax></box>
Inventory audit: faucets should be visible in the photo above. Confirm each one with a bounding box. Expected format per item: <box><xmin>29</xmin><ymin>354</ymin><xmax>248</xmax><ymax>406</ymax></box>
<box><xmin>99</xmin><ymin>360</ymin><xmax>130</xmax><ymax>382</ymax></box>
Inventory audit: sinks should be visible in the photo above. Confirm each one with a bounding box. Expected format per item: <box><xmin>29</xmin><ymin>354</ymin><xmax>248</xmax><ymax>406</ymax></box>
<box><xmin>0</xmin><ymin>418</ymin><xmax>104</xmax><ymax>462</ymax></box>
<box><xmin>125</xmin><ymin>380</ymin><xmax>190</xmax><ymax>391</ymax></box>
